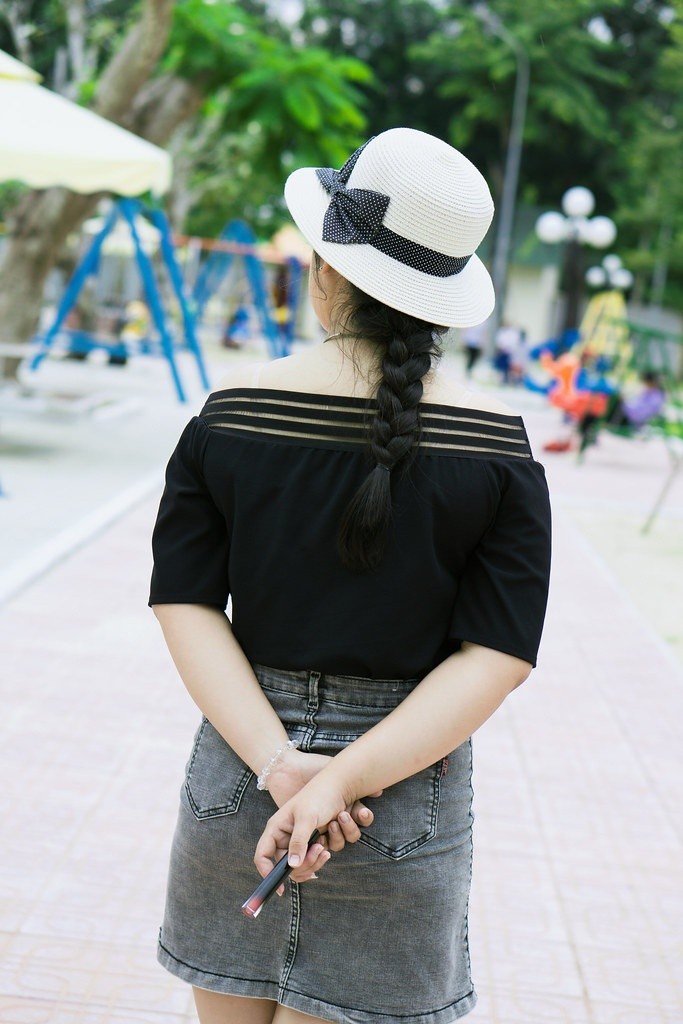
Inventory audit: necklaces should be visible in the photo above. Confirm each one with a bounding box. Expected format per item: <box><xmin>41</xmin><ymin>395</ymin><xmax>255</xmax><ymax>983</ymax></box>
<box><xmin>323</xmin><ymin>331</ymin><xmax>379</xmax><ymax>343</ymax></box>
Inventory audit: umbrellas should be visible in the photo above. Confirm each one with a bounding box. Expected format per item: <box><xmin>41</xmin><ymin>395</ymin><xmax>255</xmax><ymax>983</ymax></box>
<box><xmin>0</xmin><ymin>48</ymin><xmax>175</xmax><ymax>201</ymax></box>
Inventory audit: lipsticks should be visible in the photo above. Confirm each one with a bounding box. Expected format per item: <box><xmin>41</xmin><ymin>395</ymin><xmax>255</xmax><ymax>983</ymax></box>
<box><xmin>240</xmin><ymin>826</ymin><xmax>322</xmax><ymax>919</ymax></box>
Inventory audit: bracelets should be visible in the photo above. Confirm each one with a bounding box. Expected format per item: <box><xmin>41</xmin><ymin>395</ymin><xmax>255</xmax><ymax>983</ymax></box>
<box><xmin>258</xmin><ymin>739</ymin><xmax>301</xmax><ymax>791</ymax></box>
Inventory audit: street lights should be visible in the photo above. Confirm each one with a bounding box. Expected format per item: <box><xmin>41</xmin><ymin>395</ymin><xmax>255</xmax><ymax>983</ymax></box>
<box><xmin>470</xmin><ymin>3</ymin><xmax>612</xmax><ymax>338</ymax></box>
<box><xmin>534</xmin><ymin>186</ymin><xmax>616</xmax><ymax>350</ymax></box>
<box><xmin>584</xmin><ymin>257</ymin><xmax>632</xmax><ymax>297</ymax></box>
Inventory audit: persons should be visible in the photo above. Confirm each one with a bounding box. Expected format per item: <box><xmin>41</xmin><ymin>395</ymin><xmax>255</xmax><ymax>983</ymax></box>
<box><xmin>61</xmin><ymin>194</ymin><xmax>666</xmax><ymax>451</ymax></box>
<box><xmin>144</xmin><ymin>127</ymin><xmax>555</xmax><ymax>1023</ymax></box>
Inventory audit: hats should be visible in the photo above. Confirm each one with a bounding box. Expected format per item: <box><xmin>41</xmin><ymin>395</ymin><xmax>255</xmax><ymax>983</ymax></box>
<box><xmin>285</xmin><ymin>127</ymin><xmax>496</xmax><ymax>328</ymax></box>
